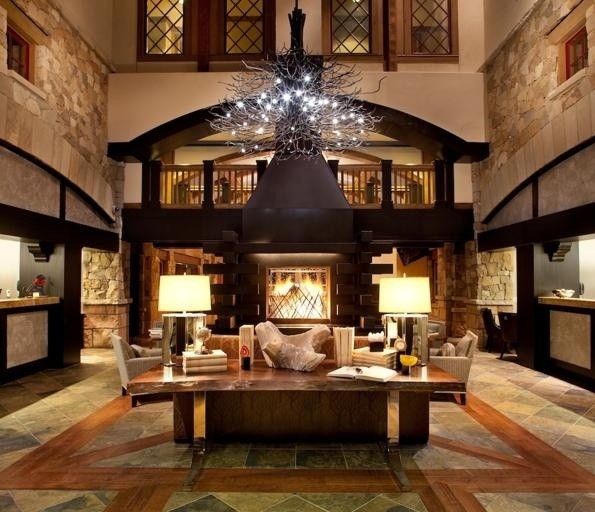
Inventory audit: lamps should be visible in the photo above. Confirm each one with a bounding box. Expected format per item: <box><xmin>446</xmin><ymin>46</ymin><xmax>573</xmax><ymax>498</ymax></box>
<box><xmin>206</xmin><ymin>45</ymin><xmax>389</xmax><ymax>168</ymax></box>
<box><xmin>155</xmin><ymin>275</ymin><xmax>212</xmax><ymax>368</ymax></box>
<box><xmin>378</xmin><ymin>274</ymin><xmax>432</xmax><ymax>365</ymax></box>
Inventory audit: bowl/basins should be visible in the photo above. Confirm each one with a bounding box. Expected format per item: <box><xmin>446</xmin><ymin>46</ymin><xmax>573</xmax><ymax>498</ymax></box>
<box><xmin>552</xmin><ymin>289</ymin><xmax>575</xmax><ymax>298</ymax></box>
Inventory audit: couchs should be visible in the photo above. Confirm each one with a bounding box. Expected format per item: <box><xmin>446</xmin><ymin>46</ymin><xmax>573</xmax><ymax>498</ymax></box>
<box><xmin>203</xmin><ymin>334</ymin><xmax>370</xmax><ymax>359</ymax></box>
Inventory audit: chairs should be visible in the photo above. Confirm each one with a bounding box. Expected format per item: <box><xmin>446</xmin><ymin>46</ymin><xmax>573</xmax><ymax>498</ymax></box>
<box><xmin>479</xmin><ymin>306</ymin><xmax>519</xmax><ymax>360</ymax></box>
<box><xmin>111</xmin><ymin>332</ymin><xmax>162</xmax><ymax>396</ymax></box>
<box><xmin>426</xmin><ymin>320</ymin><xmax>479</xmax><ymax>407</ymax></box>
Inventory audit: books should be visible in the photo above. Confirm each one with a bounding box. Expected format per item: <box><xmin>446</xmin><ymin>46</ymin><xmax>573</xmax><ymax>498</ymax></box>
<box><xmin>148</xmin><ymin>327</ymin><xmax>163</xmax><ymax>340</ymax></box>
<box><xmin>238</xmin><ymin>325</ymin><xmax>255</xmax><ymax>367</ymax></box>
<box><xmin>182</xmin><ymin>349</ymin><xmax>228</xmax><ymax>374</ymax></box>
<box><xmin>332</xmin><ymin>326</ymin><xmax>397</xmax><ymax>370</ymax></box>
<box><xmin>327</xmin><ymin>365</ymin><xmax>399</xmax><ymax>383</ymax></box>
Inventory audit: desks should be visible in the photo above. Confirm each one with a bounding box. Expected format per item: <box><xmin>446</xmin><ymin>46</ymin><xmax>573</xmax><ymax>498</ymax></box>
<box><xmin>125</xmin><ymin>353</ymin><xmax>466</xmax><ymax>470</ymax></box>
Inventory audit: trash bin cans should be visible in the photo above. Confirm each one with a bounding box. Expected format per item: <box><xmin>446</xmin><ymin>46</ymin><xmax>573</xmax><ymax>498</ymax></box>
<box><xmin>223</xmin><ymin>180</ymin><xmax>230</xmax><ymax>203</ymax></box>
<box><xmin>172</xmin><ymin>181</ymin><xmax>188</xmax><ymax>206</ymax></box>
<box><xmin>365</xmin><ymin>180</ymin><xmax>375</xmax><ymax>204</ymax></box>
<box><xmin>408</xmin><ymin>184</ymin><xmax>422</xmax><ymax>204</ymax></box>
